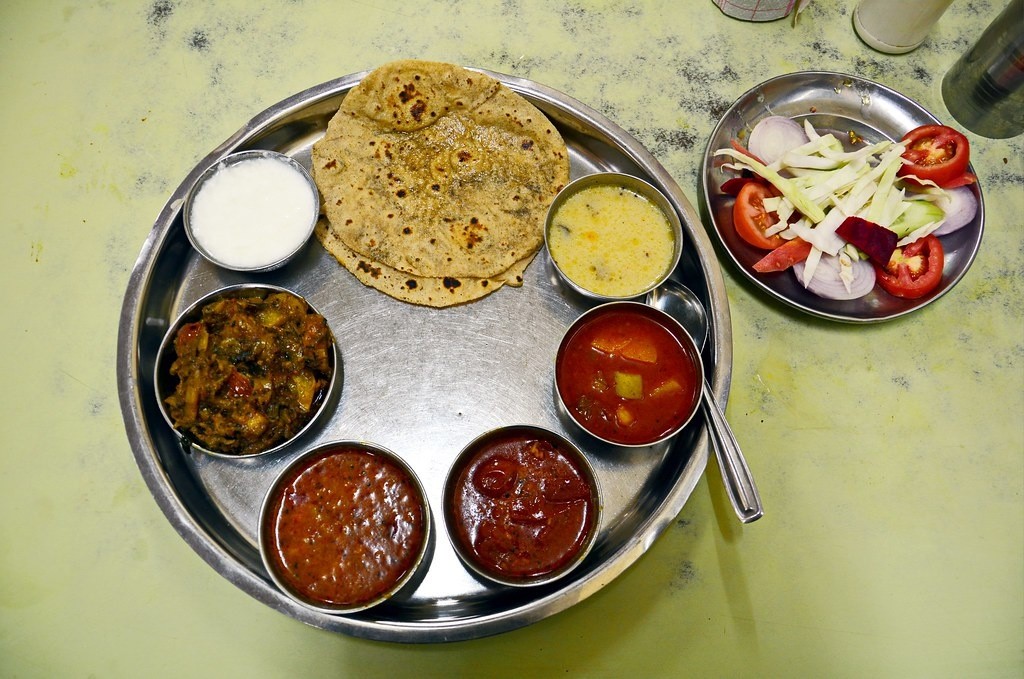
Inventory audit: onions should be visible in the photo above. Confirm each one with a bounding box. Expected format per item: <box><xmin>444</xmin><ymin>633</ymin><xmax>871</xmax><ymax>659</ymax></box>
<box><xmin>928</xmin><ymin>184</ymin><xmax>979</xmax><ymax>239</ymax></box>
<box><xmin>794</xmin><ymin>245</ymin><xmax>877</xmax><ymax>301</ymax></box>
<box><xmin>746</xmin><ymin>114</ymin><xmax>808</xmax><ymax>165</ymax></box>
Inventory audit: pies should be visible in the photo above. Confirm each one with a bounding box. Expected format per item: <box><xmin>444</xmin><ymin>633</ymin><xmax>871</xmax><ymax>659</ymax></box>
<box><xmin>303</xmin><ymin>61</ymin><xmax>576</xmax><ymax>310</ymax></box>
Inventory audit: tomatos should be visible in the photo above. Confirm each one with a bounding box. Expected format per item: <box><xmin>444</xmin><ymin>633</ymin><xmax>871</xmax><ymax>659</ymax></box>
<box><xmin>895</xmin><ymin>124</ymin><xmax>972</xmax><ymax>187</ymax></box>
<box><xmin>751</xmin><ymin>238</ymin><xmax>813</xmax><ymax>278</ymax></box>
<box><xmin>735</xmin><ymin>182</ymin><xmax>787</xmax><ymax>249</ymax></box>
<box><xmin>877</xmin><ymin>237</ymin><xmax>944</xmax><ymax>301</ymax></box>
